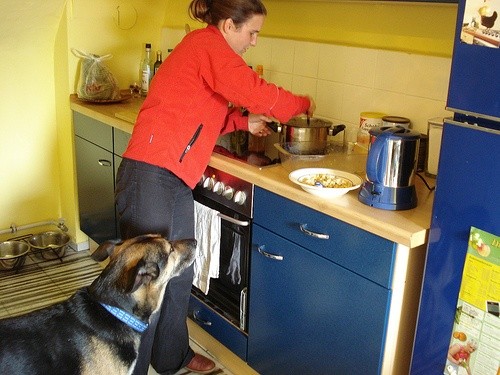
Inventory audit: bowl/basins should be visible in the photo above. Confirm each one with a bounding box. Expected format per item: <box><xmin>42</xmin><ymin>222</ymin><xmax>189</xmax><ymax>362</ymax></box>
<box><xmin>0</xmin><ymin>240</ymin><xmax>30</xmax><ymax>271</ymax></box>
<box><xmin>288</xmin><ymin>167</ymin><xmax>363</xmax><ymax>198</ymax></box>
<box><xmin>28</xmin><ymin>230</ymin><xmax>72</xmax><ymax>259</ymax></box>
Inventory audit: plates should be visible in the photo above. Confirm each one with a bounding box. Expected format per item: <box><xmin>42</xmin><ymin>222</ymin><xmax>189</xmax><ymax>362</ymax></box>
<box><xmin>84</xmin><ymin>93</ymin><xmax>132</xmax><ymax>103</ymax></box>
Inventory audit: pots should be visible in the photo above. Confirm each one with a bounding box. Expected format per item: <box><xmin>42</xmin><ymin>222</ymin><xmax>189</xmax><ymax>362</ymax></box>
<box><xmin>264</xmin><ymin>114</ymin><xmax>346</xmax><ymax>154</ymax></box>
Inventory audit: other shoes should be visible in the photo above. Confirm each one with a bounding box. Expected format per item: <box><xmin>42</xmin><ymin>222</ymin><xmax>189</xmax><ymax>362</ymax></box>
<box><xmin>184</xmin><ymin>353</ymin><xmax>216</xmax><ymax>373</ymax></box>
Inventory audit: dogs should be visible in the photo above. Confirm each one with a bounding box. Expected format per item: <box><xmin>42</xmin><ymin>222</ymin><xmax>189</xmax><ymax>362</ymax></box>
<box><xmin>0</xmin><ymin>233</ymin><xmax>197</xmax><ymax>375</ymax></box>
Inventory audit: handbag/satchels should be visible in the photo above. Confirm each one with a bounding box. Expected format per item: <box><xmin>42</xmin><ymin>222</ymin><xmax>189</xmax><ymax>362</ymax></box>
<box><xmin>73</xmin><ymin>48</ymin><xmax>120</xmax><ymax>100</ymax></box>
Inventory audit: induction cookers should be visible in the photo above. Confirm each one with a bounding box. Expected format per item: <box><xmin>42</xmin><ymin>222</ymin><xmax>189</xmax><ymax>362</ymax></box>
<box><xmin>194</xmin><ymin>130</ymin><xmax>281</xmax><ymax>218</ymax></box>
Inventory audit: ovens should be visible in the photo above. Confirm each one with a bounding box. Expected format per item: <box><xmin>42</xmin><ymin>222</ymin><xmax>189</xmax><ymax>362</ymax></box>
<box><xmin>190</xmin><ymin>189</ymin><xmax>251</xmax><ymax>332</ymax></box>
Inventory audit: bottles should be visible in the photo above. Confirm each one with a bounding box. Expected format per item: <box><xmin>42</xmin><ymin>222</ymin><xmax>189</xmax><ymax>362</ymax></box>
<box><xmin>167</xmin><ymin>48</ymin><xmax>173</xmax><ymax>56</ymax></box>
<box><xmin>241</xmin><ymin>65</ymin><xmax>263</xmax><ymax>116</ymax></box>
<box><xmin>230</xmin><ymin>129</ymin><xmax>267</xmax><ymax>153</ymax></box>
<box><xmin>140</xmin><ymin>43</ymin><xmax>153</xmax><ymax>95</ymax></box>
<box><xmin>358</xmin><ymin>111</ymin><xmax>410</xmax><ymax>145</ymax></box>
<box><xmin>154</xmin><ymin>50</ymin><xmax>163</xmax><ymax>74</ymax></box>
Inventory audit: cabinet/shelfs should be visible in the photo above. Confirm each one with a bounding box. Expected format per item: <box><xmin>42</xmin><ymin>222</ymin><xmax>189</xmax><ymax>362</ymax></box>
<box><xmin>72</xmin><ymin>111</ymin><xmax>134</xmax><ymax>255</ymax></box>
<box><xmin>188</xmin><ymin>184</ymin><xmax>426</xmax><ymax>375</ymax></box>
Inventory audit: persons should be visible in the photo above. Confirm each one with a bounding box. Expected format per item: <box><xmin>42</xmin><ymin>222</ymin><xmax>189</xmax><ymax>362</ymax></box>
<box><xmin>113</xmin><ymin>0</ymin><xmax>316</xmax><ymax>375</ymax></box>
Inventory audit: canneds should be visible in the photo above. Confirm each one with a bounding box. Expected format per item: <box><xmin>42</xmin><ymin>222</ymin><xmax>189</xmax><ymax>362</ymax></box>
<box><xmin>358</xmin><ymin>112</ymin><xmax>410</xmax><ymax>146</ymax></box>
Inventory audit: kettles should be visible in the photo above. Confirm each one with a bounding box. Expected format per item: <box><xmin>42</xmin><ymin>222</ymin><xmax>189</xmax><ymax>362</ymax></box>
<box><xmin>358</xmin><ymin>125</ymin><xmax>421</xmax><ymax>210</ymax></box>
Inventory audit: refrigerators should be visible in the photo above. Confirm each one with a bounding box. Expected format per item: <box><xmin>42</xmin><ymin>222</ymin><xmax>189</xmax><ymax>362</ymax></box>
<box><xmin>408</xmin><ymin>0</ymin><xmax>500</xmax><ymax>375</ymax></box>
